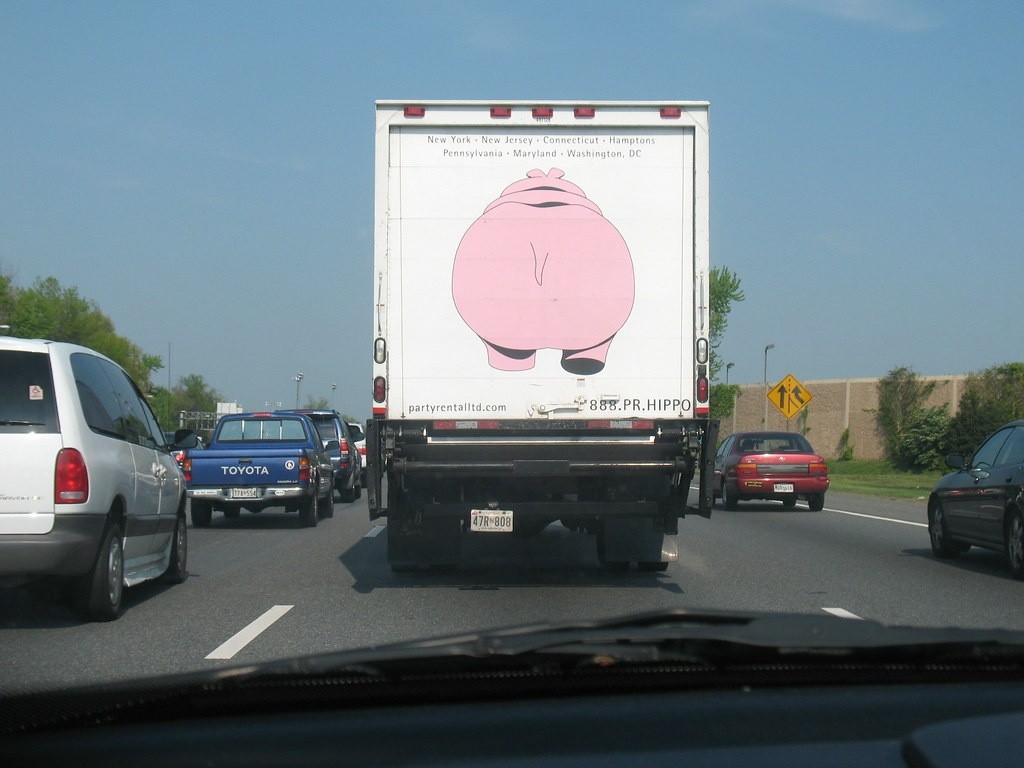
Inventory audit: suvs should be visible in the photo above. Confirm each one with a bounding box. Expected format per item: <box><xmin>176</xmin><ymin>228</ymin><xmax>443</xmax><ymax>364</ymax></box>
<box><xmin>273</xmin><ymin>409</ymin><xmax>365</xmax><ymax>504</ymax></box>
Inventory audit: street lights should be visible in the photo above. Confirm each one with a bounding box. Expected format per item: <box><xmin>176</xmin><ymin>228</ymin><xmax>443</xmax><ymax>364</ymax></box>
<box><xmin>329</xmin><ymin>383</ymin><xmax>336</xmax><ymax>409</ymax></box>
<box><xmin>295</xmin><ymin>372</ymin><xmax>305</xmax><ymax>411</ymax></box>
<box><xmin>763</xmin><ymin>343</ymin><xmax>776</xmax><ymax>431</ymax></box>
<box><xmin>726</xmin><ymin>362</ymin><xmax>734</xmax><ymax>386</ymax></box>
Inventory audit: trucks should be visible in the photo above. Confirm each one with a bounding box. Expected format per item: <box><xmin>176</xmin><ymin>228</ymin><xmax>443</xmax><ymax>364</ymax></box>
<box><xmin>369</xmin><ymin>98</ymin><xmax>714</xmax><ymax>572</ymax></box>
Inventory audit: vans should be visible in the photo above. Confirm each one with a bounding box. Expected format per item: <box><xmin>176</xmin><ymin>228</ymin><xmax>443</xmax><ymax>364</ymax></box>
<box><xmin>0</xmin><ymin>336</ymin><xmax>199</xmax><ymax>620</ymax></box>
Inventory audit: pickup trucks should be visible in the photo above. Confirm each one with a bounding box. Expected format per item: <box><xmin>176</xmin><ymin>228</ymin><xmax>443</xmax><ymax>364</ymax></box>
<box><xmin>177</xmin><ymin>412</ymin><xmax>340</xmax><ymax>527</ymax></box>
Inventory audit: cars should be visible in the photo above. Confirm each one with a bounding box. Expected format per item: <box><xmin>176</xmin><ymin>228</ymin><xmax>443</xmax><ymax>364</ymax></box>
<box><xmin>347</xmin><ymin>423</ymin><xmax>368</xmax><ymax>471</ymax></box>
<box><xmin>142</xmin><ymin>432</ymin><xmax>204</xmax><ymax>457</ymax></box>
<box><xmin>926</xmin><ymin>418</ymin><xmax>1024</xmax><ymax>579</ymax></box>
<box><xmin>715</xmin><ymin>430</ymin><xmax>830</xmax><ymax>512</ymax></box>
